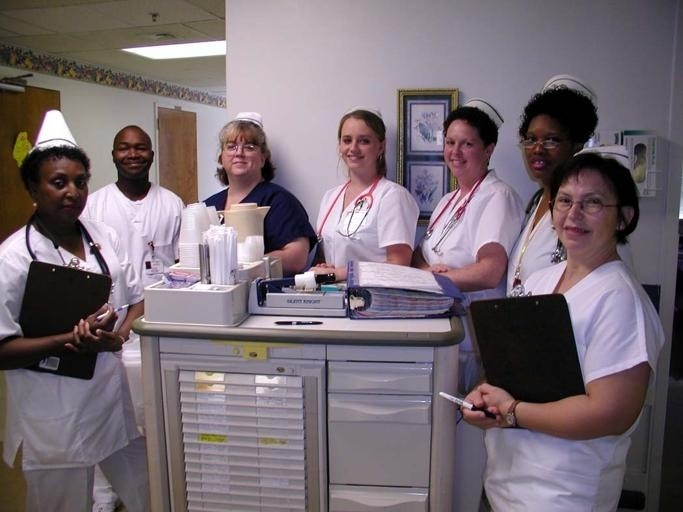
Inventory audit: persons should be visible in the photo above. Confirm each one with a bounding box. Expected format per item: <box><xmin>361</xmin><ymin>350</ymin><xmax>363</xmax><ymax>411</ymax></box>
<box><xmin>306</xmin><ymin>110</ymin><xmax>420</xmax><ymax>281</ymax></box>
<box><xmin>460</xmin><ymin>153</ymin><xmax>665</xmax><ymax>512</ymax></box>
<box><xmin>504</xmin><ymin>73</ymin><xmax>632</xmax><ymax>303</ymax></box>
<box><xmin>413</xmin><ymin>99</ymin><xmax>525</xmax><ymax>512</ymax></box>
<box><xmin>198</xmin><ymin>113</ymin><xmax>321</xmax><ymax>276</ymax></box>
<box><xmin>0</xmin><ymin>143</ymin><xmax>150</xmax><ymax>511</ymax></box>
<box><xmin>85</xmin><ymin>123</ymin><xmax>188</xmax><ymax>510</ymax></box>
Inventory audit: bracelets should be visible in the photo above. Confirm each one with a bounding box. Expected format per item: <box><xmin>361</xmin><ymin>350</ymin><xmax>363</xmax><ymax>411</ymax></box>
<box><xmin>116</xmin><ymin>334</ymin><xmax>126</xmax><ymax>356</ymax></box>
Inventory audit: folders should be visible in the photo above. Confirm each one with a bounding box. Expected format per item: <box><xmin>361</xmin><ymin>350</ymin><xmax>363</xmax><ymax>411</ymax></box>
<box><xmin>347</xmin><ymin>259</ymin><xmax>468</xmax><ymax>318</ymax></box>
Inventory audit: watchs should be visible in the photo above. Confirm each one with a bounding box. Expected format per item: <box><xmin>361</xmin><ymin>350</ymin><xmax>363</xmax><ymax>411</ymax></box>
<box><xmin>504</xmin><ymin>395</ymin><xmax>520</xmax><ymax>435</ymax></box>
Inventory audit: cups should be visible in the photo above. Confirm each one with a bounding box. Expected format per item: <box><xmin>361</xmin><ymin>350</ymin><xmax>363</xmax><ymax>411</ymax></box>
<box><xmin>178</xmin><ymin>202</ymin><xmax>222</xmax><ymax>266</ymax></box>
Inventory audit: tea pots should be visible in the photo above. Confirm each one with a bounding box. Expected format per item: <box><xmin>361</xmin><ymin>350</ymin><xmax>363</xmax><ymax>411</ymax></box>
<box><xmin>216</xmin><ymin>203</ymin><xmax>270</xmax><ymax>262</ymax></box>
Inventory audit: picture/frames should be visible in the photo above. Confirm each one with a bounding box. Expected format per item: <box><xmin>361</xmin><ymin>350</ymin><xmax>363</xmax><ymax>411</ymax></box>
<box><xmin>396</xmin><ymin>90</ymin><xmax>458</xmax><ymax>225</ymax></box>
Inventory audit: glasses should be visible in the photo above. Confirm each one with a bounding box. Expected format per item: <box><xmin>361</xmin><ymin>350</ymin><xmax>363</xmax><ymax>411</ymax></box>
<box><xmin>222</xmin><ymin>142</ymin><xmax>257</xmax><ymax>150</ymax></box>
<box><xmin>548</xmin><ymin>197</ymin><xmax>617</xmax><ymax>212</ymax></box>
<box><xmin>520</xmin><ymin>140</ymin><xmax>560</xmax><ymax>149</ymax></box>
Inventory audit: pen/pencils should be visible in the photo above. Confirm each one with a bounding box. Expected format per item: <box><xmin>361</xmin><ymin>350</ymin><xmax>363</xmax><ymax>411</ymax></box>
<box><xmin>439</xmin><ymin>391</ymin><xmax>496</xmax><ymax>420</ymax></box>
<box><xmin>96</xmin><ymin>304</ymin><xmax>129</xmax><ymax>320</ymax></box>
<box><xmin>149</xmin><ymin>241</ymin><xmax>154</xmax><ymax>250</ymax></box>
<box><xmin>276</xmin><ymin>320</ymin><xmax>323</xmax><ymax>324</ymax></box>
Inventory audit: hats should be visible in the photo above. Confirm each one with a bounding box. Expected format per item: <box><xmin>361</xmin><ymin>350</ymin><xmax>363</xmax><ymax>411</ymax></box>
<box><xmin>463</xmin><ymin>97</ymin><xmax>504</xmax><ymax>129</ymax></box>
<box><xmin>28</xmin><ymin>109</ymin><xmax>78</xmax><ymax>152</ymax></box>
<box><xmin>345</xmin><ymin>105</ymin><xmax>382</xmax><ymax>120</ymax></box>
<box><xmin>541</xmin><ymin>74</ymin><xmax>597</xmax><ymax>110</ymax></box>
<box><xmin>235</xmin><ymin>112</ymin><xmax>264</xmax><ymax>129</ymax></box>
<box><xmin>573</xmin><ymin>144</ymin><xmax>631</xmax><ymax>170</ymax></box>
